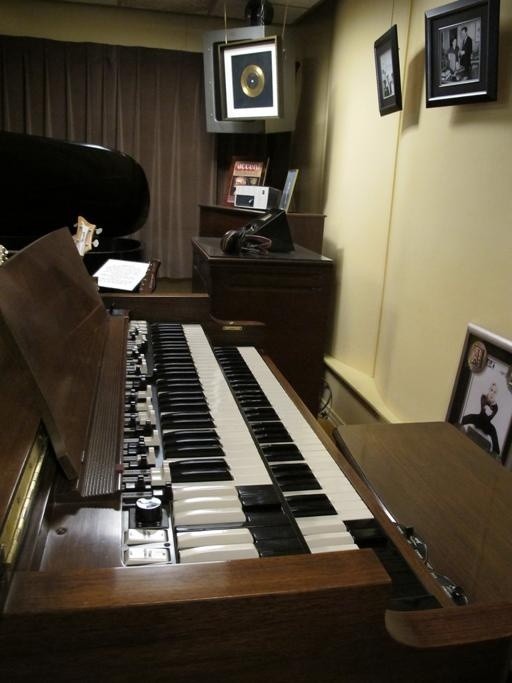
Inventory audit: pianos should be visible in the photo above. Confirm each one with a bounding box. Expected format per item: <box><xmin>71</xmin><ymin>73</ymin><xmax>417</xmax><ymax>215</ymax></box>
<box><xmin>1</xmin><ymin>227</ymin><xmax>511</xmax><ymax>683</ymax></box>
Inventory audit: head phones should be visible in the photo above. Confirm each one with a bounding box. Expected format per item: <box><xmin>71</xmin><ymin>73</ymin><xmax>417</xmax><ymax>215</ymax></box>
<box><xmin>220</xmin><ymin>228</ymin><xmax>273</xmax><ymax>255</ymax></box>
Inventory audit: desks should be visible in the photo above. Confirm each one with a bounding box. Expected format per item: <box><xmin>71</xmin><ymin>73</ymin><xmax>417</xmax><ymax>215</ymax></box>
<box><xmin>190</xmin><ymin>235</ymin><xmax>335</xmax><ymax>420</ymax></box>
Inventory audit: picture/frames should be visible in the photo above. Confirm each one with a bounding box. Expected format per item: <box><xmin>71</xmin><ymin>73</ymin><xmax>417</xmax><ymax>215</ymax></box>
<box><xmin>443</xmin><ymin>321</ymin><xmax>512</xmax><ymax>471</ymax></box>
<box><xmin>421</xmin><ymin>0</ymin><xmax>499</xmax><ymax>108</ymax></box>
<box><xmin>372</xmin><ymin>21</ymin><xmax>406</xmax><ymax>116</ymax></box>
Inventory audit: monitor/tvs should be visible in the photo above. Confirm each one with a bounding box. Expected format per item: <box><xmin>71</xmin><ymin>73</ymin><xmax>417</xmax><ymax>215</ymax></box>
<box><xmin>201</xmin><ymin>25</ymin><xmax>304</xmax><ymax>136</ymax></box>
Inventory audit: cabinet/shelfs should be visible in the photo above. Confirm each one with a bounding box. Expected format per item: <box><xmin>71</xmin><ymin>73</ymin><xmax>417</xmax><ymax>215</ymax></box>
<box><xmin>197</xmin><ymin>201</ymin><xmax>327</xmax><ymax>255</ymax></box>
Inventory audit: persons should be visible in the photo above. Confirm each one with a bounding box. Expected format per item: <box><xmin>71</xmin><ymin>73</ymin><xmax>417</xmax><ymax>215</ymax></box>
<box><xmin>456</xmin><ymin>25</ymin><xmax>473</xmax><ymax>64</ymax></box>
<box><xmin>442</xmin><ymin>35</ymin><xmax>458</xmax><ymax>71</ymax></box>
<box><xmin>463</xmin><ymin>381</ymin><xmax>502</xmax><ymax>455</ymax></box>
